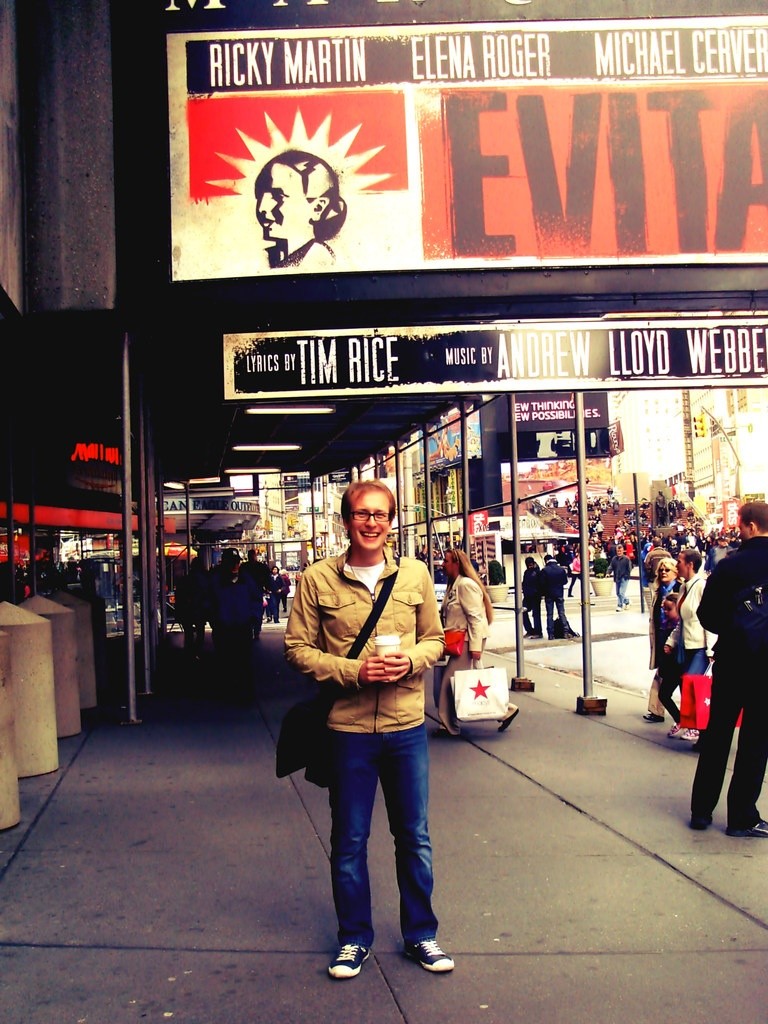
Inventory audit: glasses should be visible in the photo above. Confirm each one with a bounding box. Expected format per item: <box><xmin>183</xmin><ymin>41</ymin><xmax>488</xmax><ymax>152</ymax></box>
<box><xmin>659</xmin><ymin>568</ymin><xmax>679</xmax><ymax>575</ymax></box>
<box><xmin>351</xmin><ymin>509</ymin><xmax>392</xmax><ymax>521</ymax></box>
<box><xmin>662</xmin><ymin>606</ymin><xmax>673</xmax><ymax>611</ymax></box>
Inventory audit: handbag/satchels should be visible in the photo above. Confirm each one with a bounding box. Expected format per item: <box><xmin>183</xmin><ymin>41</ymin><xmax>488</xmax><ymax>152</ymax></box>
<box><xmin>451</xmin><ymin>657</ymin><xmax>509</xmax><ymax>722</ymax></box>
<box><xmin>433</xmin><ymin>665</ymin><xmax>447</xmax><ymax>708</ymax></box>
<box><xmin>443</xmin><ymin>629</ymin><xmax>467</xmax><ymax>655</ymax></box>
<box><xmin>275</xmin><ymin>687</ymin><xmax>340</xmax><ymax>789</ymax></box>
<box><xmin>680</xmin><ymin>662</ymin><xmax>743</xmax><ymax>729</ymax></box>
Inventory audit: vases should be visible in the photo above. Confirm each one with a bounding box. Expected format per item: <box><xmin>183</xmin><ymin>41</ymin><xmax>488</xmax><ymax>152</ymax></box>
<box><xmin>643</xmin><ymin>587</ymin><xmax>652</xmax><ymax>609</ymax></box>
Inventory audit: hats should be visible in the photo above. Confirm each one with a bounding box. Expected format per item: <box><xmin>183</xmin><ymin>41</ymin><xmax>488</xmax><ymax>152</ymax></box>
<box><xmin>223</xmin><ymin>547</ymin><xmax>244</xmax><ymax>560</ymax></box>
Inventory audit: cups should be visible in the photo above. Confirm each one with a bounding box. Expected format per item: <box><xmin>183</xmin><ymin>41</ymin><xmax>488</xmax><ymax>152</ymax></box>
<box><xmin>374</xmin><ymin>635</ymin><xmax>402</xmax><ymax>684</ymax></box>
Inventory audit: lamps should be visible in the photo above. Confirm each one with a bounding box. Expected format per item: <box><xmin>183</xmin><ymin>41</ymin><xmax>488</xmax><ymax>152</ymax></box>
<box><xmin>244</xmin><ymin>404</ymin><xmax>337</xmax><ymax>414</ymax></box>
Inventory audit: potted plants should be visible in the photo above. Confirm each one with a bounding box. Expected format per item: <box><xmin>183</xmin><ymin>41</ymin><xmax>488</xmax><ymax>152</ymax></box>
<box><xmin>589</xmin><ymin>559</ymin><xmax>614</xmax><ymax>596</ymax></box>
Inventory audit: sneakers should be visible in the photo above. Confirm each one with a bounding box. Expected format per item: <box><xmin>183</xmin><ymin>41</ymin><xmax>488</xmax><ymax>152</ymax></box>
<box><xmin>680</xmin><ymin>727</ymin><xmax>700</xmax><ymax>740</ymax></box>
<box><xmin>328</xmin><ymin>942</ymin><xmax>370</xmax><ymax>978</ymax></box>
<box><xmin>403</xmin><ymin>937</ymin><xmax>454</xmax><ymax>971</ymax></box>
<box><xmin>667</xmin><ymin>722</ymin><xmax>684</xmax><ymax>736</ymax></box>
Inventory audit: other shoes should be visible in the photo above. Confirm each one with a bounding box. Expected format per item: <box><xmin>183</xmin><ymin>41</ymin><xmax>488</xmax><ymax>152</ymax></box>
<box><xmin>431</xmin><ymin>729</ymin><xmax>452</xmax><ymax>738</ymax></box>
<box><xmin>725</xmin><ymin>819</ymin><xmax>768</xmax><ymax>838</ymax></box>
<box><xmin>563</xmin><ymin>634</ymin><xmax>573</xmax><ymax>638</ymax></box>
<box><xmin>689</xmin><ymin>815</ymin><xmax>712</xmax><ymax>830</ymax></box>
<box><xmin>531</xmin><ymin>633</ymin><xmax>542</xmax><ymax>639</ymax></box>
<box><xmin>497</xmin><ymin>709</ymin><xmax>519</xmax><ymax>732</ymax></box>
<box><xmin>643</xmin><ymin>713</ymin><xmax>664</xmax><ymax>722</ymax></box>
<box><xmin>524</xmin><ymin>633</ymin><xmax>532</xmax><ymax>638</ymax></box>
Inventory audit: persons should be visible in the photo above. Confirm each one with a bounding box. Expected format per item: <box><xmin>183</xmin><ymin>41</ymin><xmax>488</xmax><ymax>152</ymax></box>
<box><xmin>282</xmin><ymin>479</ymin><xmax>455</xmax><ymax>978</ymax></box>
<box><xmin>640</xmin><ymin>543</ymin><xmax>653</xmax><ymax>588</ymax></box>
<box><xmin>433</xmin><ymin>548</ymin><xmax>520</xmax><ymax>740</ymax></box>
<box><xmin>541</xmin><ymin>554</ymin><xmax>573</xmax><ymax>640</ymax></box>
<box><xmin>688</xmin><ymin>501</ymin><xmax>768</xmax><ymax>838</ymax></box>
<box><xmin>644</xmin><ymin>536</ymin><xmax>672</xmax><ymax>583</ymax></box>
<box><xmin>532</xmin><ymin>484</ymin><xmax>742</xmax><ymax>576</ymax></box>
<box><xmin>663</xmin><ymin>549</ymin><xmax>718</xmax><ymax>753</ymax></box>
<box><xmin>608</xmin><ymin>545</ymin><xmax>631</xmax><ymax>612</ymax></box>
<box><xmin>656</xmin><ymin>491</ymin><xmax>666</xmax><ymax>528</ymax></box>
<box><xmin>522</xmin><ymin>557</ymin><xmax>543</xmax><ymax>639</ymax></box>
<box><xmin>643</xmin><ymin>557</ymin><xmax>685</xmax><ymax>722</ymax></box>
<box><xmin>417</xmin><ymin>546</ymin><xmax>479</xmax><ymax>584</ymax></box>
<box><xmin>175</xmin><ymin>548</ymin><xmax>291</xmax><ymax>705</ymax></box>
<box><xmin>658</xmin><ymin>593</ymin><xmax>699</xmax><ymax>740</ymax></box>
<box><xmin>568</xmin><ymin>551</ymin><xmax>593</xmax><ymax>597</ymax></box>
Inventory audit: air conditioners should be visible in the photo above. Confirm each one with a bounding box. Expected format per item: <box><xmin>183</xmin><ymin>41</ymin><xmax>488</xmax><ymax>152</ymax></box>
<box><xmin>484</xmin><ymin>560</ymin><xmax>510</xmax><ymax>603</ymax></box>
